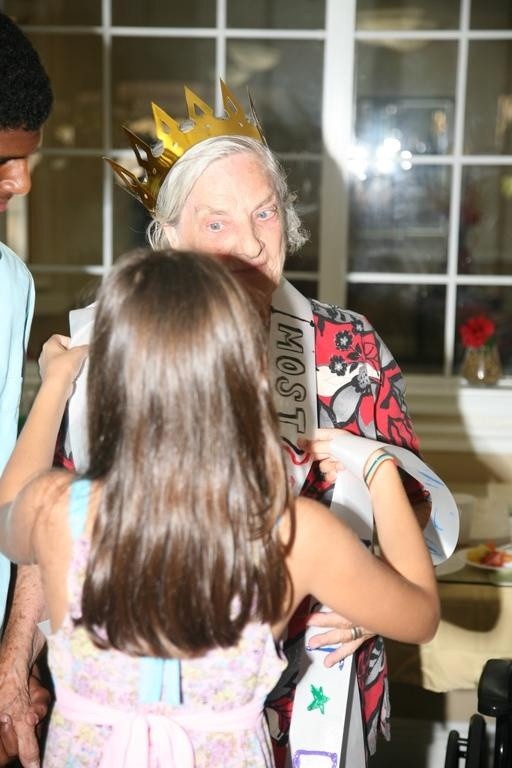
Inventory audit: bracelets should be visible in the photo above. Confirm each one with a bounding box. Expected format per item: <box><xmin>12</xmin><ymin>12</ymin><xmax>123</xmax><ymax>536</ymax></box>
<box><xmin>364</xmin><ymin>452</ymin><xmax>399</xmax><ymax>487</ymax></box>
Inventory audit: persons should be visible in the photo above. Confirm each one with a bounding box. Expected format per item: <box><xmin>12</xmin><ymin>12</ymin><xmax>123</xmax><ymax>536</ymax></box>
<box><xmin>0</xmin><ymin>248</ymin><xmax>443</xmax><ymax>768</ymax></box>
<box><xmin>0</xmin><ymin>11</ymin><xmax>55</xmax><ymax>765</ymax></box>
<box><xmin>1</xmin><ymin>113</ymin><xmax>431</xmax><ymax>768</ymax></box>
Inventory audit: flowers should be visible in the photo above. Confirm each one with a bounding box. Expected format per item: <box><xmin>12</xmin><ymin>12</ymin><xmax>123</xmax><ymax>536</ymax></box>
<box><xmin>460</xmin><ymin>314</ymin><xmax>497</xmax><ymax>354</ymax></box>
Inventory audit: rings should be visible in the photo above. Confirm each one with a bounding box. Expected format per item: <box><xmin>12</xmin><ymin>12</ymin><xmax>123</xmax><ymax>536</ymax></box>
<box><xmin>349</xmin><ymin>625</ymin><xmax>362</xmax><ymax>641</ymax></box>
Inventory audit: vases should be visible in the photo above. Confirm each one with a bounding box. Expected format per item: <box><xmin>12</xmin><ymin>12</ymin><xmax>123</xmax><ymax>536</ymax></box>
<box><xmin>455</xmin><ymin>344</ymin><xmax>504</xmax><ymax>386</ymax></box>
